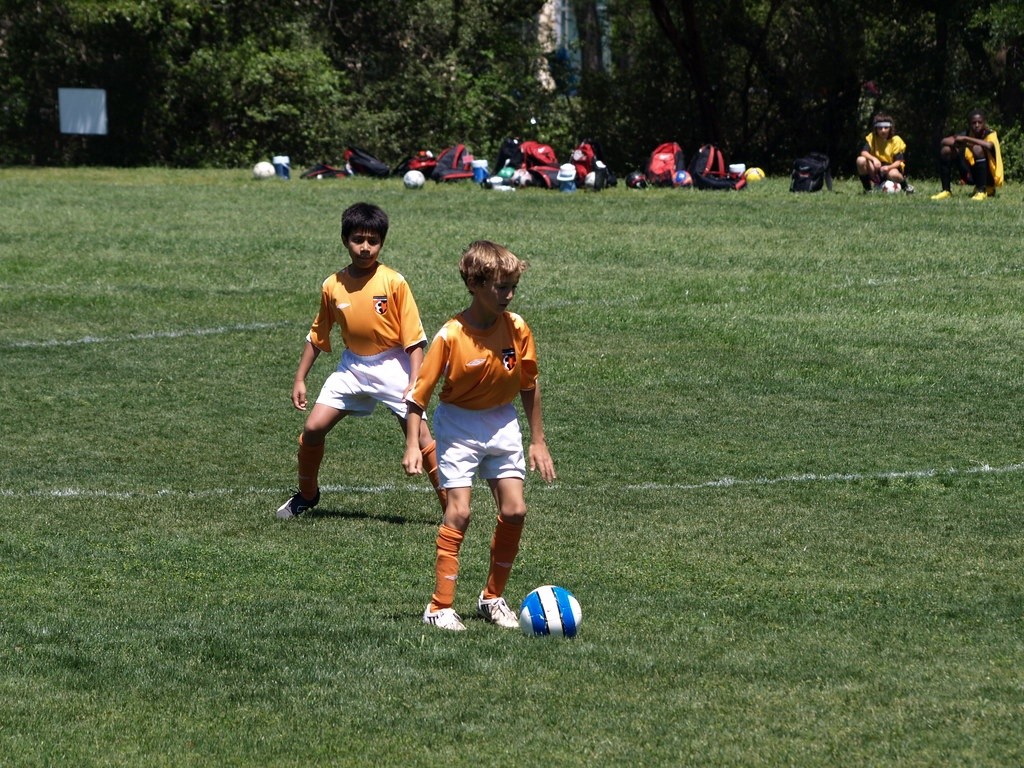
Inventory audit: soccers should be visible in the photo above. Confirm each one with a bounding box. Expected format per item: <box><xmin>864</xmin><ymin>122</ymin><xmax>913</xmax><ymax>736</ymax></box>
<box><xmin>974</xmin><ymin>185</ymin><xmax>996</xmax><ymax>197</ymax></box>
<box><xmin>403</xmin><ymin>169</ymin><xmax>425</xmax><ymax>190</ymax></box>
<box><xmin>626</xmin><ymin>172</ymin><xmax>646</xmax><ymax>189</ymax></box>
<box><xmin>743</xmin><ymin>167</ymin><xmax>765</xmax><ymax>184</ymax></box>
<box><xmin>670</xmin><ymin>170</ymin><xmax>693</xmax><ymax>189</ymax></box>
<box><xmin>584</xmin><ymin>171</ymin><xmax>606</xmax><ymax>189</ymax></box>
<box><xmin>520</xmin><ymin>585</ymin><xmax>582</xmax><ymax>637</ymax></box>
<box><xmin>881</xmin><ymin>180</ymin><xmax>901</xmax><ymax>193</ymax></box>
<box><xmin>253</xmin><ymin>162</ymin><xmax>276</xmax><ymax>179</ymax></box>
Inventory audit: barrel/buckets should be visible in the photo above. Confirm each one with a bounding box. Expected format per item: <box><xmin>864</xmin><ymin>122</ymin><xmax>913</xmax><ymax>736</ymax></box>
<box><xmin>273</xmin><ymin>156</ymin><xmax>291</xmax><ymax>180</ymax></box>
<box><xmin>556</xmin><ymin>162</ymin><xmax>577</xmax><ymax>193</ymax></box>
<box><xmin>471</xmin><ymin>159</ymin><xmax>490</xmax><ymax>185</ymax></box>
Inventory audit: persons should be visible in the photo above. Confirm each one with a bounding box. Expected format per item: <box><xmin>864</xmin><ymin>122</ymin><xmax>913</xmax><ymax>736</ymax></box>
<box><xmin>276</xmin><ymin>203</ymin><xmax>447</xmax><ymax>519</ymax></box>
<box><xmin>930</xmin><ymin>112</ymin><xmax>1003</xmax><ymax>200</ymax></box>
<box><xmin>856</xmin><ymin>114</ymin><xmax>915</xmax><ymax>195</ymax></box>
<box><xmin>402</xmin><ymin>240</ymin><xmax>557</xmax><ymax>628</ymax></box>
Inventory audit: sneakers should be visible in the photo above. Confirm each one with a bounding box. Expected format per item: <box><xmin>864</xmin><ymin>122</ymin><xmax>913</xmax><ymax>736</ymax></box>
<box><xmin>476</xmin><ymin>590</ymin><xmax>520</xmax><ymax>629</ymax></box>
<box><xmin>971</xmin><ymin>191</ymin><xmax>987</xmax><ymax>201</ymax></box>
<box><xmin>904</xmin><ymin>184</ymin><xmax>914</xmax><ymax>193</ymax></box>
<box><xmin>275</xmin><ymin>486</ymin><xmax>320</xmax><ymax>521</ymax></box>
<box><xmin>422</xmin><ymin>604</ymin><xmax>466</xmax><ymax>631</ymax></box>
<box><xmin>931</xmin><ymin>190</ymin><xmax>952</xmax><ymax>202</ymax></box>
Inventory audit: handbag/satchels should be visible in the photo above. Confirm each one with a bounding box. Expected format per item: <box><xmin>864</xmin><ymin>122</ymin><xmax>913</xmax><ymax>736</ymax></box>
<box><xmin>788</xmin><ymin>153</ymin><xmax>832</xmax><ymax>193</ymax></box>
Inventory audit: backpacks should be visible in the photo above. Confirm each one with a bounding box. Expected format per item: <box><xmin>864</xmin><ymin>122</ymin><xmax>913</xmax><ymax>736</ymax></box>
<box><xmin>392</xmin><ymin>150</ymin><xmax>437</xmax><ymax>178</ymax></box>
<box><xmin>687</xmin><ymin>143</ymin><xmax>725</xmax><ymax>184</ymax></box>
<box><xmin>569</xmin><ymin>138</ymin><xmax>617</xmax><ymax>188</ymax></box>
<box><xmin>693</xmin><ymin>171</ymin><xmax>746</xmax><ymax>191</ymax></box>
<box><xmin>300</xmin><ymin>162</ymin><xmax>346</xmax><ymax>179</ymax></box>
<box><xmin>343</xmin><ymin>145</ymin><xmax>389</xmax><ymax>179</ymax></box>
<box><xmin>488</xmin><ymin>138</ymin><xmax>524</xmax><ymax>178</ymax></box>
<box><xmin>430</xmin><ymin>144</ymin><xmax>474</xmax><ymax>183</ymax></box>
<box><xmin>519</xmin><ymin>141</ymin><xmax>561</xmax><ymax>190</ymax></box>
<box><xmin>647</xmin><ymin>143</ymin><xmax>685</xmax><ymax>186</ymax></box>
